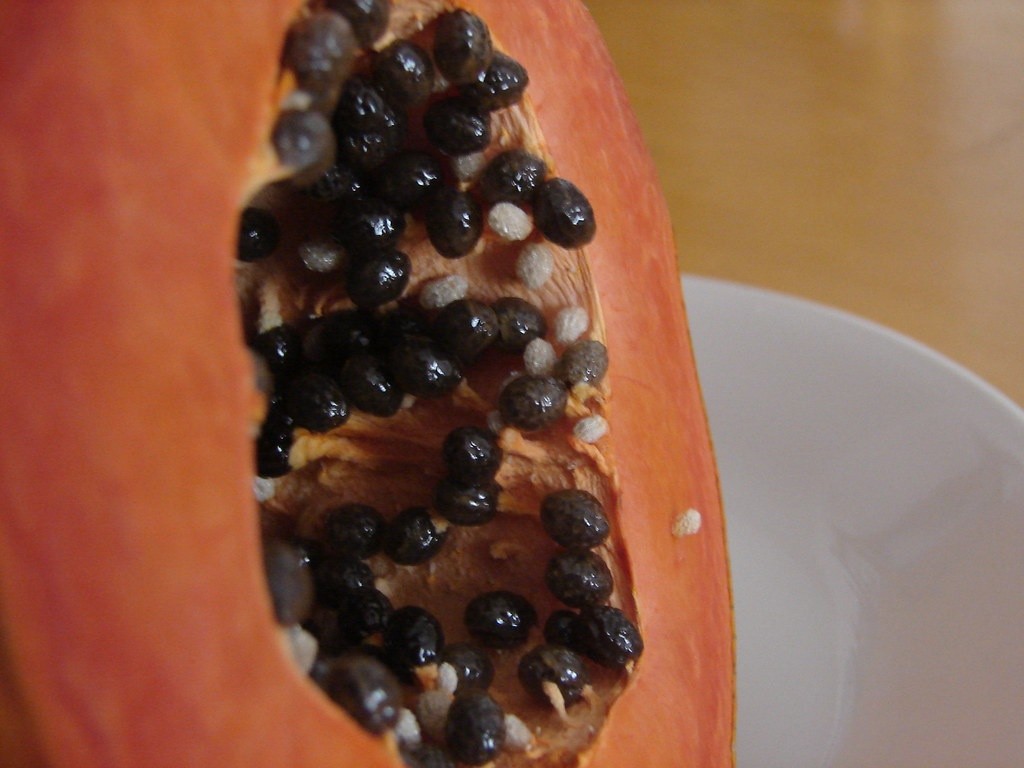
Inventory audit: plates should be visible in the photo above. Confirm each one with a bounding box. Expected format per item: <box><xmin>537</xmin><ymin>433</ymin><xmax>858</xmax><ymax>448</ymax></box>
<box><xmin>679</xmin><ymin>272</ymin><xmax>1024</xmax><ymax>768</ymax></box>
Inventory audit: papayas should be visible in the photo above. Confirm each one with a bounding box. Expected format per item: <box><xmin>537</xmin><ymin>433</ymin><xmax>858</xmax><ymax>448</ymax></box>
<box><xmin>0</xmin><ymin>0</ymin><xmax>735</xmax><ymax>768</ymax></box>
